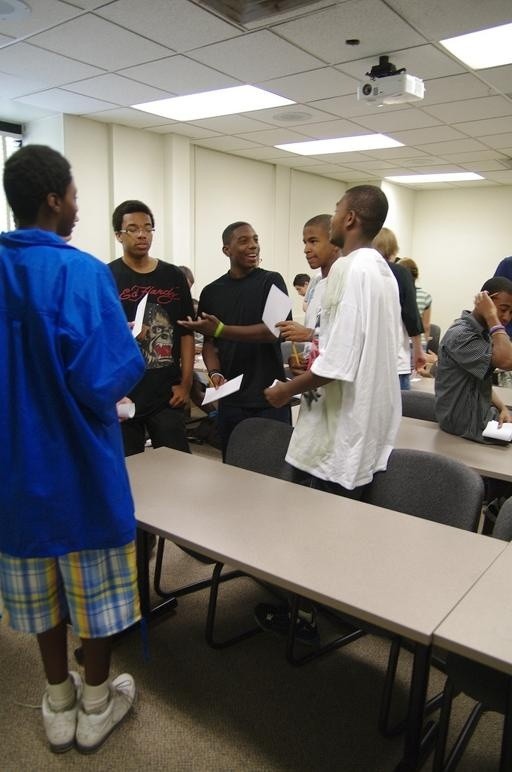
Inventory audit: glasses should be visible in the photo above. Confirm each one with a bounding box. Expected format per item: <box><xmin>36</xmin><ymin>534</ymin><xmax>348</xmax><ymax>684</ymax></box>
<box><xmin>119</xmin><ymin>226</ymin><xmax>156</xmax><ymax>235</ymax></box>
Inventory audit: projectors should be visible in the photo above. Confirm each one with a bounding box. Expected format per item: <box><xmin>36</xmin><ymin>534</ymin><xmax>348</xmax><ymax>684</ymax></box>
<box><xmin>356</xmin><ymin>74</ymin><xmax>427</xmax><ymax>109</ymax></box>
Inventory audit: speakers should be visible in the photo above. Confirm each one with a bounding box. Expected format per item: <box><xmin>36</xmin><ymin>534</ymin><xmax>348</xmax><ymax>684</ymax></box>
<box><xmin>0</xmin><ymin>119</ymin><xmax>26</xmax><ymax>138</ymax></box>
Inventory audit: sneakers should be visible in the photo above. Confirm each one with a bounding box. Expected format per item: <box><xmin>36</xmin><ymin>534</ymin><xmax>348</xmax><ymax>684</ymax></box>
<box><xmin>75</xmin><ymin>673</ymin><xmax>136</xmax><ymax>754</ymax></box>
<box><xmin>42</xmin><ymin>670</ymin><xmax>81</xmax><ymax>753</ymax></box>
<box><xmin>253</xmin><ymin>602</ymin><xmax>319</xmax><ymax>645</ymax></box>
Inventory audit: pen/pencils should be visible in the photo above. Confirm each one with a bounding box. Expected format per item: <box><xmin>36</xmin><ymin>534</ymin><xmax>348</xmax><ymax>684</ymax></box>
<box><xmin>207</xmin><ymin>377</ymin><xmax>213</xmax><ymax>387</ymax></box>
<box><xmin>489</xmin><ymin>293</ymin><xmax>497</xmax><ymax>297</ymax></box>
<box><xmin>292</xmin><ymin>345</ymin><xmax>300</xmax><ymax>364</ymax></box>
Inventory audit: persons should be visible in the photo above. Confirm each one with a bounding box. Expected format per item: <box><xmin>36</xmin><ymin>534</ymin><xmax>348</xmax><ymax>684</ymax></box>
<box><xmin>103</xmin><ymin>180</ymin><xmax>511</xmax><ymax>650</ymax></box>
<box><xmin>57</xmin><ymin>215</ymin><xmax>80</xmax><ymax>244</ymax></box>
<box><xmin>0</xmin><ymin>141</ymin><xmax>154</xmax><ymax>757</ymax></box>
